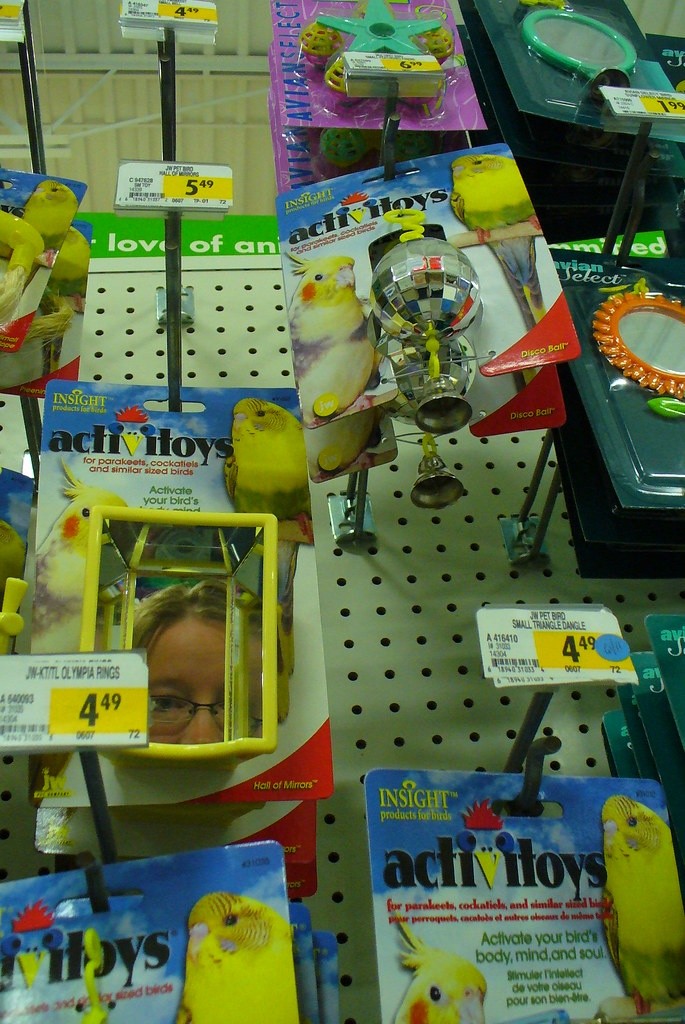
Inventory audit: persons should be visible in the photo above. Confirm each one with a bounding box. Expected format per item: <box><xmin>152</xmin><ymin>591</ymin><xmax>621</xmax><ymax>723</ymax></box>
<box><xmin>113</xmin><ymin>580</ymin><xmax>283</xmax><ymax>806</ymax></box>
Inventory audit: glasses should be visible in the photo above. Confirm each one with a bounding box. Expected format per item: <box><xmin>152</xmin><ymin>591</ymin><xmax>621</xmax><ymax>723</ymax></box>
<box><xmin>149</xmin><ymin>695</ymin><xmax>263</xmax><ymax>732</ymax></box>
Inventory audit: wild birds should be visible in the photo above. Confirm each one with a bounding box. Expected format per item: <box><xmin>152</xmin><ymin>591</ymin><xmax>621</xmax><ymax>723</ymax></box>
<box><xmin>389</xmin><ymin>912</ymin><xmax>493</xmax><ymax>1024</ymax></box>
<box><xmin>287</xmin><ymin>249</ymin><xmax>373</xmax><ymax>474</ymax></box>
<box><xmin>599</xmin><ymin>791</ymin><xmax>684</xmax><ymax>1017</ymax></box>
<box><xmin>22</xmin><ymin>178</ymin><xmax>89</xmax><ymax>304</ymax></box>
<box><xmin>448</xmin><ymin>153</ymin><xmax>547</xmax><ymax>392</ymax></box>
<box><xmin>221</xmin><ymin>391</ymin><xmax>312</xmax><ymax>735</ymax></box>
<box><xmin>166</xmin><ymin>891</ymin><xmax>302</xmax><ymax>1024</ymax></box>
<box><xmin>30</xmin><ymin>454</ymin><xmax>133</xmax><ymax>659</ymax></box>
